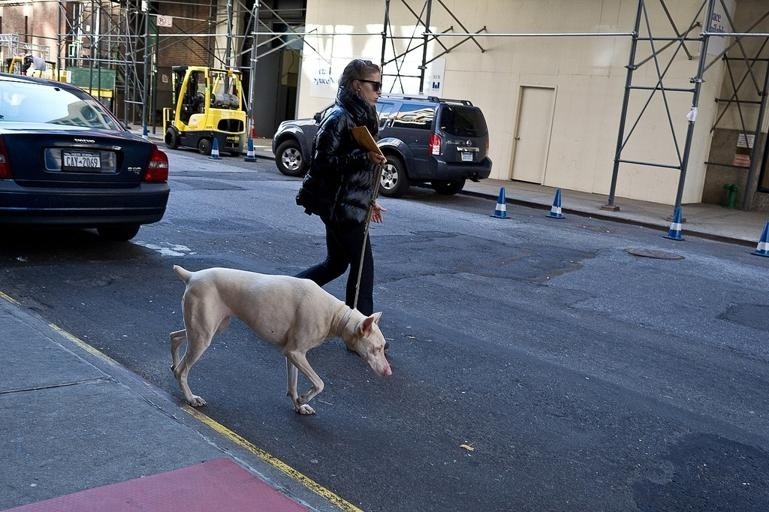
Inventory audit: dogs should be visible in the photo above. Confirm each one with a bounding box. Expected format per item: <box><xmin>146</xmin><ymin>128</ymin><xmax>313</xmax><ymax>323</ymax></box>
<box><xmin>168</xmin><ymin>264</ymin><xmax>393</xmax><ymax>416</ymax></box>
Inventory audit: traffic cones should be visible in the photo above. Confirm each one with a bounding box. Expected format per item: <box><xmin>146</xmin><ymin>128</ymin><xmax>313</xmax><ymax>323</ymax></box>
<box><xmin>207</xmin><ymin>137</ymin><xmax>222</xmax><ymax>159</ymax></box>
<box><xmin>244</xmin><ymin>136</ymin><xmax>258</xmax><ymax>161</ymax></box>
<box><xmin>749</xmin><ymin>221</ymin><xmax>769</xmax><ymax>257</ymax></box>
<box><xmin>490</xmin><ymin>187</ymin><xmax>512</xmax><ymax>219</ymax></box>
<box><xmin>545</xmin><ymin>188</ymin><xmax>567</xmax><ymax>219</ymax></box>
<box><xmin>662</xmin><ymin>206</ymin><xmax>686</xmax><ymax>241</ymax></box>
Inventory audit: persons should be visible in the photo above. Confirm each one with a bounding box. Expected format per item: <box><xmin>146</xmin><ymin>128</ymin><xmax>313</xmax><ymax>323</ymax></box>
<box><xmin>22</xmin><ymin>56</ymin><xmax>35</xmax><ymax>77</ymax></box>
<box><xmin>292</xmin><ymin>59</ymin><xmax>390</xmax><ymax>355</ymax></box>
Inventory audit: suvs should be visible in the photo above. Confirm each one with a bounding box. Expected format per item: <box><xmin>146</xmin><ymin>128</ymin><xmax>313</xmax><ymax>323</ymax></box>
<box><xmin>272</xmin><ymin>91</ymin><xmax>492</xmax><ymax>197</ymax></box>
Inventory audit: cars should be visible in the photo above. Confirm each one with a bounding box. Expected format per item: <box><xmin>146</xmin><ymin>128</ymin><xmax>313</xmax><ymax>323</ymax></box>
<box><xmin>0</xmin><ymin>73</ymin><xmax>171</xmax><ymax>245</ymax></box>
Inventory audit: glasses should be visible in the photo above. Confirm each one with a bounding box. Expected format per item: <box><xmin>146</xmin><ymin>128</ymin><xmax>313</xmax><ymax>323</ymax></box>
<box><xmin>351</xmin><ymin>77</ymin><xmax>383</xmax><ymax>91</ymax></box>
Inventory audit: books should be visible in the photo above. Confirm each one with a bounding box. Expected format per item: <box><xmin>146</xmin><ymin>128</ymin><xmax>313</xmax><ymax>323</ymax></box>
<box><xmin>352</xmin><ymin>125</ymin><xmax>387</xmax><ymax>163</ymax></box>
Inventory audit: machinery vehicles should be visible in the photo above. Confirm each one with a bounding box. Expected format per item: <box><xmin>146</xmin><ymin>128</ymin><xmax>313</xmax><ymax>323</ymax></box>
<box><xmin>6</xmin><ymin>55</ymin><xmax>117</xmax><ymax>111</ymax></box>
<box><xmin>163</xmin><ymin>64</ymin><xmax>247</xmax><ymax>157</ymax></box>
